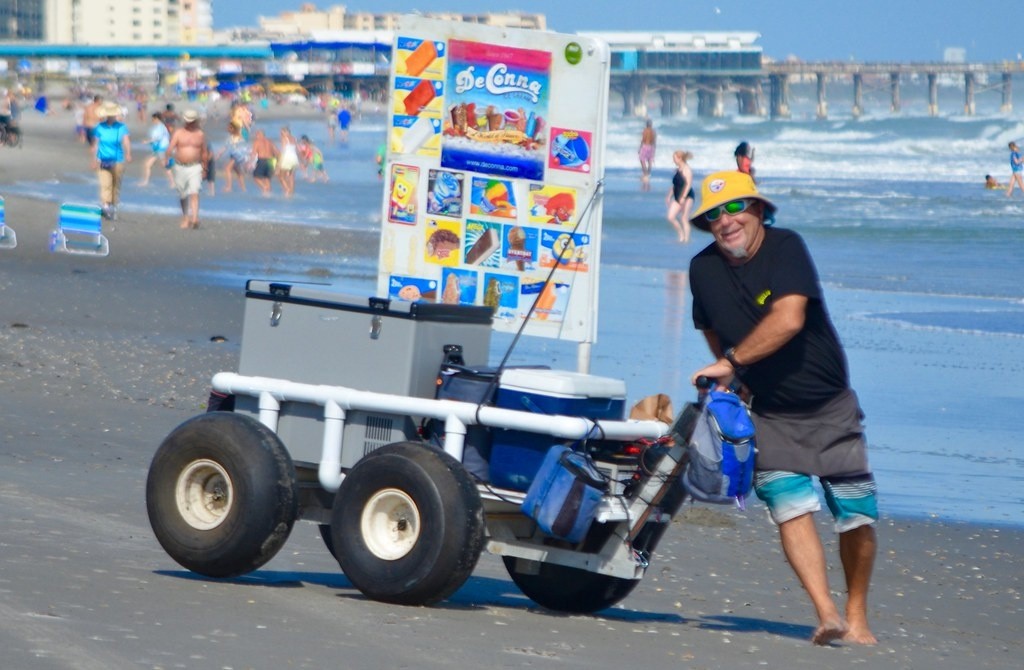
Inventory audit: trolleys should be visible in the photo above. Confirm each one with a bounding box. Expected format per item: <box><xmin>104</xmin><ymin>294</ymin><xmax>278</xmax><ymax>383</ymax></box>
<box><xmin>145</xmin><ymin>277</ymin><xmax>717</xmax><ymax>609</ymax></box>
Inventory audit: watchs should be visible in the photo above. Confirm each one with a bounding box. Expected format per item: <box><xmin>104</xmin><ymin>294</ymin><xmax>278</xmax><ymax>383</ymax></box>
<box><xmin>725</xmin><ymin>346</ymin><xmax>743</xmax><ymax>369</ymax></box>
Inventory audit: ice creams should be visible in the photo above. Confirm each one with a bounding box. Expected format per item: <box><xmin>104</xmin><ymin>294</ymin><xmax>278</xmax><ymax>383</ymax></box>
<box><xmin>508</xmin><ymin>228</ymin><xmax>527</xmax><ymax>271</ymax></box>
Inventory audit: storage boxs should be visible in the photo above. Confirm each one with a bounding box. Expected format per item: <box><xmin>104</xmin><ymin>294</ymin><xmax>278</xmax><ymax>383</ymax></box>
<box><xmin>492</xmin><ymin>368</ymin><xmax>627</xmax><ymax>492</ymax></box>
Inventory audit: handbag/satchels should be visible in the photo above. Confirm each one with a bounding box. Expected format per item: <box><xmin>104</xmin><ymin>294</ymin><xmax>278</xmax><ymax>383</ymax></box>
<box><xmin>681</xmin><ymin>384</ymin><xmax>757</xmax><ymax>507</ymax></box>
<box><xmin>520</xmin><ymin>442</ymin><xmax>608</xmax><ymax>545</ymax></box>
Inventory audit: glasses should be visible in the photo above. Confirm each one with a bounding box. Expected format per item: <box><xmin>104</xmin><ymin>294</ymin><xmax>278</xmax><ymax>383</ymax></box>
<box><xmin>705</xmin><ymin>199</ymin><xmax>755</xmax><ymax>221</ymax></box>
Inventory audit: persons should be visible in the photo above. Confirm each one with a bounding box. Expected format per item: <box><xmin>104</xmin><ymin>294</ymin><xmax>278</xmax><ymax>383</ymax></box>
<box><xmin>667</xmin><ymin>151</ymin><xmax>695</xmax><ymax>243</ymax></box>
<box><xmin>985</xmin><ymin>175</ymin><xmax>999</xmax><ymax>189</ymax></box>
<box><xmin>638</xmin><ymin>120</ymin><xmax>656</xmax><ymax>182</ymax></box>
<box><xmin>688</xmin><ymin>171</ymin><xmax>883</xmax><ymax>646</ymax></box>
<box><xmin>735</xmin><ymin>141</ymin><xmax>757</xmax><ymax>186</ymax></box>
<box><xmin>376</xmin><ymin>135</ymin><xmax>387</xmax><ymax>180</ymax></box>
<box><xmin>1005</xmin><ymin>142</ymin><xmax>1024</xmax><ymax>196</ymax></box>
<box><xmin>1</xmin><ymin>69</ymin><xmax>361</xmax><ymax>229</ymax></box>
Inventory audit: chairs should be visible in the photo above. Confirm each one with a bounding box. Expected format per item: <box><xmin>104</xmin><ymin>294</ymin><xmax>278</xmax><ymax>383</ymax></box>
<box><xmin>0</xmin><ymin>195</ymin><xmax>17</xmax><ymax>249</ymax></box>
<box><xmin>50</xmin><ymin>201</ymin><xmax>109</xmax><ymax>257</ymax></box>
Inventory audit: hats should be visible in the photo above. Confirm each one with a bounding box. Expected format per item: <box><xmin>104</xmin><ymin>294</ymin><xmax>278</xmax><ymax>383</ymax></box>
<box><xmin>97</xmin><ymin>101</ymin><xmax>123</xmax><ymax>117</ymax></box>
<box><xmin>183</xmin><ymin>110</ymin><xmax>197</xmax><ymax>124</ymax></box>
<box><xmin>689</xmin><ymin>171</ymin><xmax>777</xmax><ymax>233</ymax></box>
